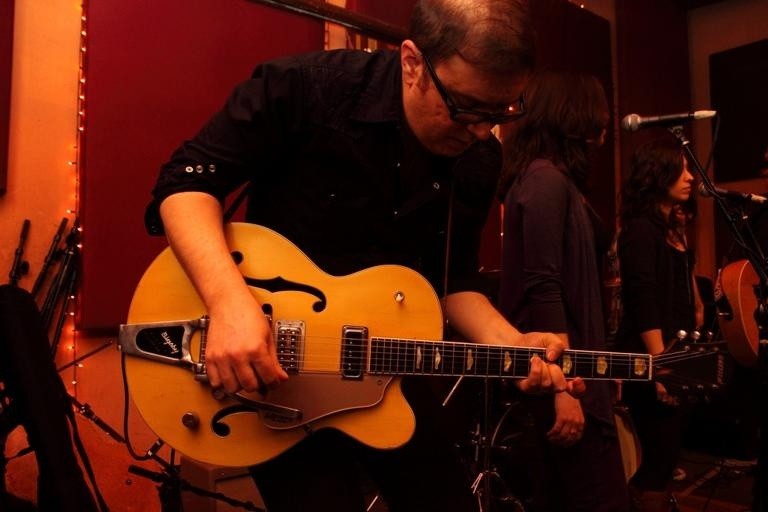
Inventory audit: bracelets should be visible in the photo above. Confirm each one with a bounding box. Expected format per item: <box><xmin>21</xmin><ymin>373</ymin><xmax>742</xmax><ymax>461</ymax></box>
<box><xmin>555</xmin><ymin>385</ymin><xmax>567</xmax><ymax>394</ymax></box>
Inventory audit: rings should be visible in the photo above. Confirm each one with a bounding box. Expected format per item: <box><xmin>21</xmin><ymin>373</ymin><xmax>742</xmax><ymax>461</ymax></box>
<box><xmin>570</xmin><ymin>432</ymin><xmax>575</xmax><ymax>435</ymax></box>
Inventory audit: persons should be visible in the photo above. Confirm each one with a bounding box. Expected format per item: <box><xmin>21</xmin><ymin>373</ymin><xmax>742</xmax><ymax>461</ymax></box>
<box><xmin>498</xmin><ymin>60</ymin><xmax>654</xmax><ymax>512</ymax></box>
<box><xmin>616</xmin><ymin>140</ymin><xmax>723</xmax><ymax>488</ymax></box>
<box><xmin>136</xmin><ymin>0</ymin><xmax>585</xmax><ymax>510</ymax></box>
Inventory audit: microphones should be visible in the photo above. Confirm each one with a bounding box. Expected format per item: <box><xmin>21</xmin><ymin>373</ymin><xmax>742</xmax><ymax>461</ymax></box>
<box><xmin>697</xmin><ymin>180</ymin><xmax>767</xmax><ymax>207</ymax></box>
<box><xmin>620</xmin><ymin>108</ymin><xmax>716</xmax><ymax>132</ymax></box>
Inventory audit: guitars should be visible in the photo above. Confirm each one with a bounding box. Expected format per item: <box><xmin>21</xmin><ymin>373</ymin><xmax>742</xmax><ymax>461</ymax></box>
<box><xmin>117</xmin><ymin>222</ymin><xmax>725</xmax><ymax>466</ymax></box>
<box><xmin>715</xmin><ymin>260</ymin><xmax>768</xmax><ymax>359</ymax></box>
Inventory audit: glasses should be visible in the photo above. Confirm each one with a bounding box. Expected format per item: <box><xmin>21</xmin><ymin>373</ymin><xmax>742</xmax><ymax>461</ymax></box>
<box><xmin>424</xmin><ymin>55</ymin><xmax>527</xmax><ymax>125</ymax></box>
